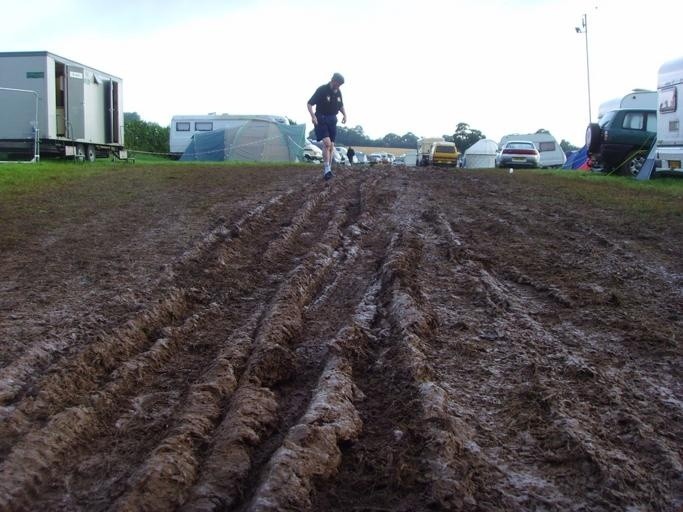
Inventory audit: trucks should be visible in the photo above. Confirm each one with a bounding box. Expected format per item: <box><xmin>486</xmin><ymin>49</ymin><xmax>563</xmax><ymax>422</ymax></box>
<box><xmin>170</xmin><ymin>115</ymin><xmax>323</xmax><ymax>162</ymax></box>
<box><xmin>416</xmin><ymin>138</ymin><xmax>446</xmax><ymax>166</ymax></box>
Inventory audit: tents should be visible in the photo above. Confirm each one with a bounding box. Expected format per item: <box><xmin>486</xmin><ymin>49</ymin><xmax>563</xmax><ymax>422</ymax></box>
<box><xmin>460</xmin><ymin>139</ymin><xmax>498</xmax><ymax>168</ymax></box>
<box><xmin>178</xmin><ymin>121</ymin><xmax>306</xmax><ymax>163</ymax></box>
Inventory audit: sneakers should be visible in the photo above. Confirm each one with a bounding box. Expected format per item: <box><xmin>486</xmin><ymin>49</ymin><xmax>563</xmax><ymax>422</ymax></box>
<box><xmin>324</xmin><ymin>170</ymin><xmax>337</xmax><ymax>179</ymax></box>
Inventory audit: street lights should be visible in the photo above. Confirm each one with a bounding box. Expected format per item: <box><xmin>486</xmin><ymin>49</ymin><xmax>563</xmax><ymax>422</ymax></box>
<box><xmin>576</xmin><ymin>13</ymin><xmax>592</xmax><ymax>124</ymax></box>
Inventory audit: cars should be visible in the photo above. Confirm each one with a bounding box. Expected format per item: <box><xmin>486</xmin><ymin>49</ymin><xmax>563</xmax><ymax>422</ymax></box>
<box><xmin>314</xmin><ymin>143</ymin><xmax>395</xmax><ymax>164</ymax></box>
<box><xmin>495</xmin><ymin>141</ymin><xmax>540</xmax><ymax>168</ymax></box>
<box><xmin>430</xmin><ymin>143</ymin><xmax>458</xmax><ymax>166</ymax></box>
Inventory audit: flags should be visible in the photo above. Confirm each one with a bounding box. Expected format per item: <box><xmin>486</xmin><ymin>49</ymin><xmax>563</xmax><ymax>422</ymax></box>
<box><xmin>574</xmin><ymin>14</ymin><xmax>586</xmax><ymax>33</ymax></box>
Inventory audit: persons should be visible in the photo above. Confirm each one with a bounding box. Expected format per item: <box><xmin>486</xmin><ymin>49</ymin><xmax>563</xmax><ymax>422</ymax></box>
<box><xmin>306</xmin><ymin>72</ymin><xmax>348</xmax><ymax>179</ymax></box>
<box><xmin>346</xmin><ymin>146</ymin><xmax>355</xmax><ymax>165</ymax></box>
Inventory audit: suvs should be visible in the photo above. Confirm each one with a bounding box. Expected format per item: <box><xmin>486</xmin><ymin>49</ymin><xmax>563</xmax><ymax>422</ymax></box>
<box><xmin>586</xmin><ymin>108</ymin><xmax>658</xmax><ymax>176</ymax></box>
